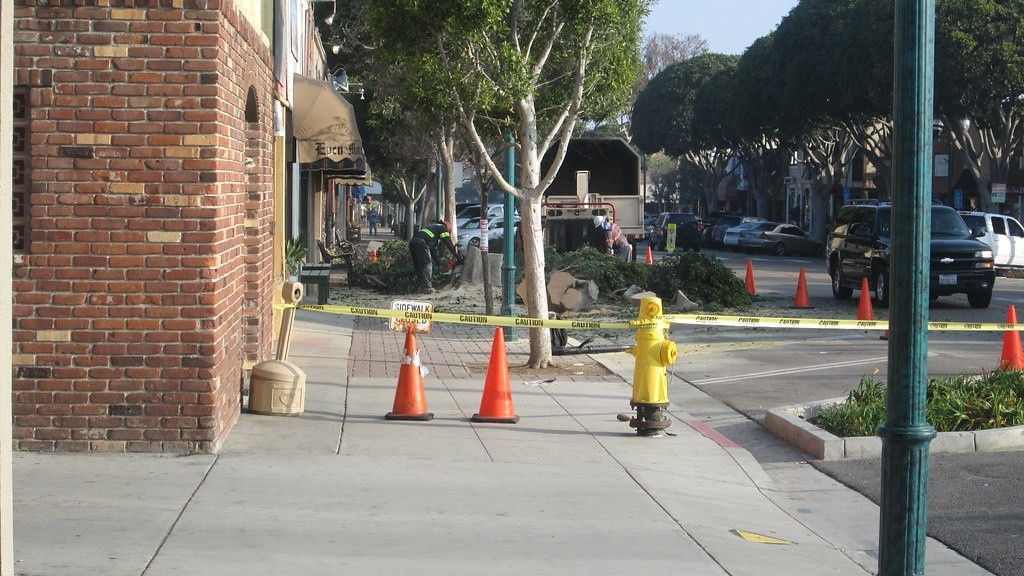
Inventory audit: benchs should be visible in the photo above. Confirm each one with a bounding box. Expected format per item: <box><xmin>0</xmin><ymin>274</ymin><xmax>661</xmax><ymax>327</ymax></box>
<box><xmin>336</xmin><ymin>228</ymin><xmax>360</xmax><ymax>253</ymax></box>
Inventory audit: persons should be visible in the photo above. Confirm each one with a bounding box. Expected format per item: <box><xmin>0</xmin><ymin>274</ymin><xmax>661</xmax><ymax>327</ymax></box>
<box><xmin>368</xmin><ymin>211</ymin><xmax>378</xmax><ymax>236</ymax></box>
<box><xmin>409</xmin><ymin>220</ymin><xmax>465</xmax><ymax>294</ymax></box>
<box><xmin>999</xmin><ymin>206</ymin><xmax>1012</xmax><ymax>216</ymax></box>
<box><xmin>367</xmin><ymin>206</ymin><xmax>379</xmax><ymax>224</ymax></box>
<box><xmin>594</xmin><ymin>216</ymin><xmax>630</xmax><ymax>261</ymax></box>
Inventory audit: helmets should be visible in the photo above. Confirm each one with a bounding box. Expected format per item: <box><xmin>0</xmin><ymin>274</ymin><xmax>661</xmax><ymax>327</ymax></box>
<box><xmin>593</xmin><ymin>216</ymin><xmax>605</xmax><ymax>229</ymax></box>
<box><xmin>443</xmin><ymin>221</ymin><xmax>452</xmax><ymax>233</ymax></box>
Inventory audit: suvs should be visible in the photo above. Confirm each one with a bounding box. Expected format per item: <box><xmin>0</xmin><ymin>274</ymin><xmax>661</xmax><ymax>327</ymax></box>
<box><xmin>456</xmin><ymin>204</ymin><xmax>519</xmax><ymax>228</ymax></box>
<box><xmin>827</xmin><ymin>198</ymin><xmax>995</xmax><ymax>307</ymax></box>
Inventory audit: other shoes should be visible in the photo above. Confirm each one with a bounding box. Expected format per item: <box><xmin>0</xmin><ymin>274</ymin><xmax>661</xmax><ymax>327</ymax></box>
<box><xmin>425</xmin><ymin>289</ymin><xmax>438</xmax><ymax>294</ymax></box>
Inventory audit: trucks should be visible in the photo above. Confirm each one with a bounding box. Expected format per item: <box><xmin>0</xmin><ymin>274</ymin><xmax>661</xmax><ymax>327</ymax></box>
<box><xmin>539</xmin><ymin>136</ymin><xmax>646</xmax><ymax>262</ymax></box>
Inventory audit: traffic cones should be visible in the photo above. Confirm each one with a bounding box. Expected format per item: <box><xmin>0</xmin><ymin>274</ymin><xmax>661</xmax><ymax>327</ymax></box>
<box><xmin>879</xmin><ymin>313</ymin><xmax>891</xmax><ymax>340</ymax></box>
<box><xmin>380</xmin><ymin>323</ymin><xmax>435</xmax><ymax>421</ymax></box>
<box><xmin>992</xmin><ymin>305</ymin><xmax>1024</xmax><ymax>371</ymax></box>
<box><xmin>469</xmin><ymin>324</ymin><xmax>520</xmax><ymax>424</ymax></box>
<box><xmin>744</xmin><ymin>258</ymin><xmax>760</xmax><ymax>296</ymax></box>
<box><xmin>646</xmin><ymin>245</ymin><xmax>654</xmax><ymax>264</ymax></box>
<box><xmin>856</xmin><ymin>278</ymin><xmax>876</xmax><ymax>321</ymax></box>
<box><xmin>788</xmin><ymin>265</ymin><xmax>823</xmax><ymax>313</ymax></box>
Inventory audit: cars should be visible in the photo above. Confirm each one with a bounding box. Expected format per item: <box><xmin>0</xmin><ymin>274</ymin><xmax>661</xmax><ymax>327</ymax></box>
<box><xmin>738</xmin><ymin>223</ymin><xmax>821</xmax><ymax>258</ymax></box>
<box><xmin>650</xmin><ymin>212</ymin><xmax>702</xmax><ymax>252</ymax></box>
<box><xmin>711</xmin><ymin>216</ymin><xmax>770</xmax><ymax>251</ymax></box>
<box><xmin>644</xmin><ymin>214</ymin><xmax>658</xmax><ymax>238</ymax></box>
<box><xmin>703</xmin><ymin>211</ymin><xmax>739</xmax><ymax>244</ymax></box>
<box><xmin>955</xmin><ymin>210</ymin><xmax>1024</xmax><ymax>271</ymax></box>
<box><xmin>696</xmin><ymin>218</ymin><xmax>705</xmax><ymax>231</ymax></box>
<box><xmin>456</xmin><ymin>216</ymin><xmax>521</xmax><ymax>258</ymax></box>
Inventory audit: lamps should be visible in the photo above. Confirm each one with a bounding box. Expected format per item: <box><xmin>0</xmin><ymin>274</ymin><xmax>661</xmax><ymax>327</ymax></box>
<box><xmin>329</xmin><ymin>63</ymin><xmax>348</xmax><ymax>91</ymax></box>
<box><xmin>322</xmin><ymin>42</ymin><xmax>342</xmax><ymax>54</ymax></box>
<box><xmin>310</xmin><ymin>0</ymin><xmax>336</xmax><ymax>25</ymax></box>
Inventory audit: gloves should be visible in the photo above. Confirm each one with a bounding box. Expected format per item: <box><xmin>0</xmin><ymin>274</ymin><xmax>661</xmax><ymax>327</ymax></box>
<box><xmin>435</xmin><ymin>259</ymin><xmax>441</xmax><ymax>267</ymax></box>
<box><xmin>457</xmin><ymin>255</ymin><xmax>464</xmax><ymax>262</ymax></box>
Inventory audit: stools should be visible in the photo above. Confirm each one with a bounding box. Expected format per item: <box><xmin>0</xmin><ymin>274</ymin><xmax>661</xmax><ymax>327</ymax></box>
<box><xmin>302</xmin><ymin>262</ymin><xmax>331</xmax><ymax>305</ymax></box>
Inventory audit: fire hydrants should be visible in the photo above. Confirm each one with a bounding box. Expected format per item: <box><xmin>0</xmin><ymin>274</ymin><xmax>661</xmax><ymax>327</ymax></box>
<box><xmin>618</xmin><ymin>292</ymin><xmax>679</xmax><ymax>437</ymax></box>
